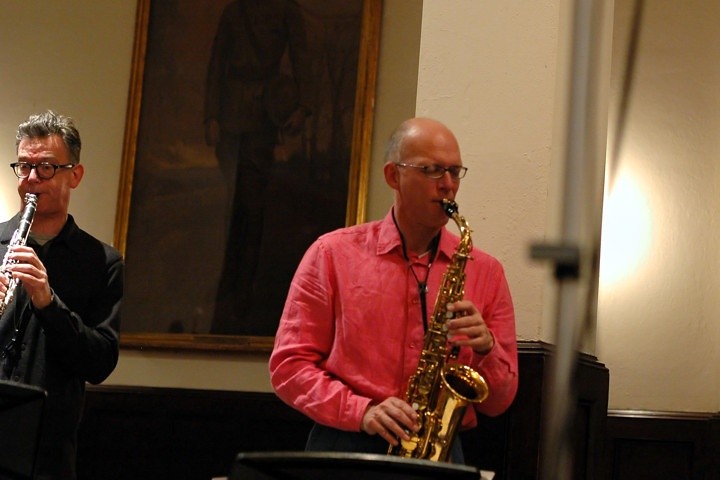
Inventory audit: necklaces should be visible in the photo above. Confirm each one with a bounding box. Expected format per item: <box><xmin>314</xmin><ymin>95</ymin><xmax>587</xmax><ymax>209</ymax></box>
<box><xmin>400</xmin><ymin>238</ymin><xmax>437</xmax><ymax>334</ymax></box>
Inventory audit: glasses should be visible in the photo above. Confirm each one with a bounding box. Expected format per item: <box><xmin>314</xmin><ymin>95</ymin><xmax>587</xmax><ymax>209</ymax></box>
<box><xmin>10</xmin><ymin>162</ymin><xmax>74</xmax><ymax>180</ymax></box>
<box><xmin>396</xmin><ymin>164</ymin><xmax>467</xmax><ymax>179</ymax></box>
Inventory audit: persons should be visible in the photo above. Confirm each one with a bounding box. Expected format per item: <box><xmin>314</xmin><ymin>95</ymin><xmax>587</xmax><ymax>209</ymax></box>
<box><xmin>269</xmin><ymin>118</ymin><xmax>518</xmax><ymax>465</ymax></box>
<box><xmin>0</xmin><ymin>109</ymin><xmax>124</xmax><ymax>480</ymax></box>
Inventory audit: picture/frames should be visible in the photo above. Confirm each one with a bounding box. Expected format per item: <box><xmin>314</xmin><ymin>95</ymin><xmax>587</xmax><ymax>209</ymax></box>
<box><xmin>113</xmin><ymin>0</ymin><xmax>385</xmax><ymax>353</ymax></box>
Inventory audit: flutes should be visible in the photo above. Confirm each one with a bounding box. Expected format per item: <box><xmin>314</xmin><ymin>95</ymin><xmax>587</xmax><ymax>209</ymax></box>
<box><xmin>0</xmin><ymin>190</ymin><xmax>39</xmax><ymax>323</ymax></box>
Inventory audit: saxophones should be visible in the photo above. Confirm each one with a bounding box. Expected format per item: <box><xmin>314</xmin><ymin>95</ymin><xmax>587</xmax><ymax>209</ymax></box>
<box><xmin>385</xmin><ymin>197</ymin><xmax>489</xmax><ymax>463</ymax></box>
